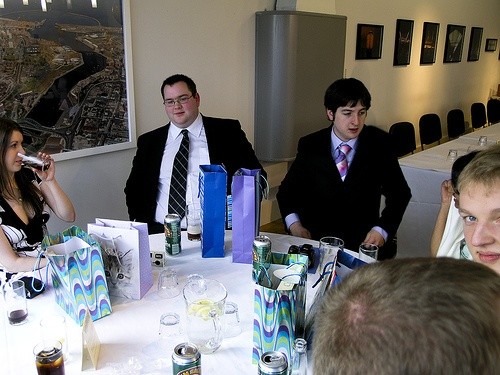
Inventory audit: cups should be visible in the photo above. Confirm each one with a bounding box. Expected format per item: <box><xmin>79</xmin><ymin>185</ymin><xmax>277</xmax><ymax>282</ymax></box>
<box><xmin>2</xmin><ymin>280</ymin><xmax>29</xmax><ymax>327</ymax></box>
<box><xmin>187</xmin><ymin>273</ymin><xmax>208</xmax><ymax>300</ymax></box>
<box><xmin>477</xmin><ymin>135</ymin><xmax>487</xmax><ymax>146</ymax></box>
<box><xmin>359</xmin><ymin>243</ymin><xmax>378</xmax><ymax>264</ymax></box>
<box><xmin>185</xmin><ymin>202</ymin><xmax>202</xmax><ymax>241</ymax></box>
<box><xmin>319</xmin><ymin>236</ymin><xmax>344</xmax><ymax>281</ymax></box>
<box><xmin>224</xmin><ymin>301</ymin><xmax>242</xmax><ymax>340</ymax></box>
<box><xmin>467</xmin><ymin>146</ymin><xmax>474</xmax><ymax>153</ymax></box>
<box><xmin>15</xmin><ymin>147</ymin><xmax>51</xmax><ymax>171</ymax></box>
<box><xmin>157</xmin><ymin>271</ymin><xmax>180</xmax><ymax>299</ymax></box>
<box><xmin>39</xmin><ymin>315</ymin><xmax>70</xmax><ymax>364</ymax></box>
<box><xmin>158</xmin><ymin>311</ymin><xmax>182</xmax><ymax>346</ymax></box>
<box><xmin>32</xmin><ymin>339</ymin><xmax>65</xmax><ymax>375</ymax></box>
<box><xmin>446</xmin><ymin>149</ymin><xmax>458</xmax><ymax>162</ymax></box>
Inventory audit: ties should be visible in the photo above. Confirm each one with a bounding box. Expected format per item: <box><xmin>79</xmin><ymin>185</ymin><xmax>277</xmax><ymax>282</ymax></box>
<box><xmin>334</xmin><ymin>144</ymin><xmax>351</xmax><ymax>181</ymax></box>
<box><xmin>168</xmin><ymin>129</ymin><xmax>189</xmax><ymax>220</ymax></box>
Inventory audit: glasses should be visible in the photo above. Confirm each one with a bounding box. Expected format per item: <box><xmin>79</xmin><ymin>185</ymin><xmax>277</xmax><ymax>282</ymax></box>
<box><xmin>163</xmin><ymin>95</ymin><xmax>193</xmax><ymax>106</ymax></box>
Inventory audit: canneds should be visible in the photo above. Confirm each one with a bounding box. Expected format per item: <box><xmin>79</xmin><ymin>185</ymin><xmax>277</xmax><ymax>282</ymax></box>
<box><xmin>171</xmin><ymin>342</ymin><xmax>202</xmax><ymax>375</ymax></box>
<box><xmin>258</xmin><ymin>351</ymin><xmax>288</xmax><ymax>375</ymax></box>
<box><xmin>251</xmin><ymin>234</ymin><xmax>272</xmax><ymax>281</ymax></box>
<box><xmin>164</xmin><ymin>213</ymin><xmax>182</xmax><ymax>257</ymax></box>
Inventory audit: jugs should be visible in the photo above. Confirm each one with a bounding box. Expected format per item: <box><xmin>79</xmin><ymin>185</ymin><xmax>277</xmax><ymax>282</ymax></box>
<box><xmin>182</xmin><ymin>277</ymin><xmax>228</xmax><ymax>356</ymax></box>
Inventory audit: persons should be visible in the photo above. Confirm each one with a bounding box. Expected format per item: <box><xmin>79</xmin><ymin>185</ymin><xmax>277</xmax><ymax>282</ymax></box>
<box><xmin>0</xmin><ymin>118</ymin><xmax>75</xmax><ymax>286</ymax></box>
<box><xmin>276</xmin><ymin>78</ymin><xmax>412</xmax><ymax>261</ymax></box>
<box><xmin>124</xmin><ymin>74</ymin><xmax>268</xmax><ymax>235</ymax></box>
<box><xmin>310</xmin><ymin>257</ymin><xmax>500</xmax><ymax>375</ymax></box>
<box><xmin>430</xmin><ymin>150</ymin><xmax>482</xmax><ymax>262</ymax></box>
<box><xmin>458</xmin><ymin>142</ymin><xmax>500</xmax><ymax>275</ymax></box>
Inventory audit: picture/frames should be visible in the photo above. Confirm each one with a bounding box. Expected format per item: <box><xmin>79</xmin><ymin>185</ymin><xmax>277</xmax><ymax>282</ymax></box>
<box><xmin>355</xmin><ymin>23</ymin><xmax>384</xmax><ymax>60</ymax></box>
<box><xmin>420</xmin><ymin>21</ymin><xmax>440</xmax><ymax>64</ymax></box>
<box><xmin>0</xmin><ymin>0</ymin><xmax>137</xmax><ymax>162</ymax></box>
<box><xmin>443</xmin><ymin>24</ymin><xmax>466</xmax><ymax>63</ymax></box>
<box><xmin>393</xmin><ymin>18</ymin><xmax>414</xmax><ymax>66</ymax></box>
<box><xmin>485</xmin><ymin>38</ymin><xmax>498</xmax><ymax>51</ymax></box>
<box><xmin>467</xmin><ymin>27</ymin><xmax>484</xmax><ymax>61</ymax></box>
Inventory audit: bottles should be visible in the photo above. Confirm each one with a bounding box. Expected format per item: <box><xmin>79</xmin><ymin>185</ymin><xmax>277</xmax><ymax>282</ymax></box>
<box><xmin>289</xmin><ymin>338</ymin><xmax>308</xmax><ymax>375</ymax></box>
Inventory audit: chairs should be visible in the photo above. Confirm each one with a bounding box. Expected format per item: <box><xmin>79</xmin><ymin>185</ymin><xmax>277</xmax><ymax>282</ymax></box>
<box><xmin>389</xmin><ymin>122</ymin><xmax>417</xmax><ymax>156</ymax></box>
<box><xmin>447</xmin><ymin>109</ymin><xmax>464</xmax><ymax>141</ymax></box>
<box><xmin>471</xmin><ymin>102</ymin><xmax>486</xmax><ymax>131</ymax></box>
<box><xmin>419</xmin><ymin>114</ymin><xmax>443</xmax><ymax>151</ymax></box>
<box><xmin>486</xmin><ymin>99</ymin><xmax>500</xmax><ymax>127</ymax></box>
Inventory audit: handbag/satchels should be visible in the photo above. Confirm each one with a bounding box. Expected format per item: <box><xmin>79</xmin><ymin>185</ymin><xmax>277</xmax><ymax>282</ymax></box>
<box><xmin>231</xmin><ymin>168</ymin><xmax>270</xmax><ymax>264</ymax></box>
<box><xmin>252</xmin><ymin>262</ymin><xmax>309</xmax><ymax>372</ymax></box>
<box><xmin>197</xmin><ymin>165</ymin><xmax>227</xmax><ymax>258</ymax></box>
<box><xmin>303</xmin><ymin>257</ymin><xmax>340</xmax><ymax>349</ymax></box>
<box><xmin>87</xmin><ymin>218</ymin><xmax>154</xmax><ymax>300</ymax></box>
<box><xmin>31</xmin><ymin>225</ymin><xmax>112</xmax><ymax>327</ymax></box>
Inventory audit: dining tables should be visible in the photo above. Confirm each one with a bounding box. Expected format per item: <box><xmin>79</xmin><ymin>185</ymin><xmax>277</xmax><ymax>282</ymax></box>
<box><xmin>0</xmin><ymin>231</ymin><xmax>376</xmax><ymax>375</ymax></box>
<box><xmin>395</xmin><ymin>122</ymin><xmax>500</xmax><ymax>257</ymax></box>
